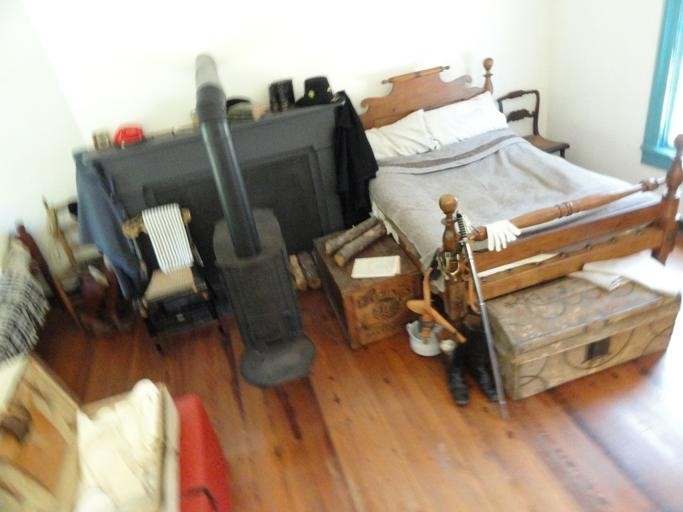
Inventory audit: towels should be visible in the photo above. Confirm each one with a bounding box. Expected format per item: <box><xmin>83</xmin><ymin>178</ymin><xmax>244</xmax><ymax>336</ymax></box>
<box><xmin>582</xmin><ymin>251</ymin><xmax>679</xmax><ymax>299</ymax></box>
<box><xmin>141</xmin><ymin>204</ymin><xmax>194</xmax><ymax>273</ymax></box>
<box><xmin>566</xmin><ymin>270</ymin><xmax>629</xmax><ymax>290</ymax></box>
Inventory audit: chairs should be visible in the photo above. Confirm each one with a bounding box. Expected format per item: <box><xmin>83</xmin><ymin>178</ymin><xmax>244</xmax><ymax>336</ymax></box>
<box><xmin>40</xmin><ymin>193</ymin><xmax>120</xmax><ymax>334</ymax></box>
<box><xmin>123</xmin><ymin>203</ymin><xmax>228</xmax><ymax>354</ymax></box>
<box><xmin>497</xmin><ymin>87</ymin><xmax>570</xmax><ymax>160</ymax></box>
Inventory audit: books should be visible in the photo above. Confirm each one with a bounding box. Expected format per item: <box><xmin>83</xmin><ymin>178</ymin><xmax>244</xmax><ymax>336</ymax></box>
<box><xmin>350</xmin><ymin>254</ymin><xmax>401</xmax><ymax>280</ymax></box>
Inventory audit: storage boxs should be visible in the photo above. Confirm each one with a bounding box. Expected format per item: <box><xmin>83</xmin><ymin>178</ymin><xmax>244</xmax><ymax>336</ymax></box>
<box><xmin>0</xmin><ymin>353</ymin><xmax>84</xmax><ymax>512</ymax></box>
<box><xmin>77</xmin><ymin>379</ymin><xmax>178</xmax><ymax>511</ymax></box>
<box><xmin>483</xmin><ymin>256</ymin><xmax>681</xmax><ymax>401</ymax></box>
<box><xmin>172</xmin><ymin>395</ymin><xmax>232</xmax><ymax>512</ymax></box>
<box><xmin>313</xmin><ymin>221</ymin><xmax>420</xmax><ymax>351</ymax></box>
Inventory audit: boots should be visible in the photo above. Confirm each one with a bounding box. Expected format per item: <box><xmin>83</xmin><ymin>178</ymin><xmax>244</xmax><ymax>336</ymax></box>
<box><xmin>448</xmin><ymin>344</ymin><xmax>469</xmax><ymax>404</ymax></box>
<box><xmin>467</xmin><ymin>331</ymin><xmax>498</xmax><ymax>402</ymax></box>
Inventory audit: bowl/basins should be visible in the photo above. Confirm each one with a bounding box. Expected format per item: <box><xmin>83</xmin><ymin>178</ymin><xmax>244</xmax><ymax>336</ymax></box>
<box><xmin>405</xmin><ymin>320</ymin><xmax>443</xmax><ymax>357</ymax></box>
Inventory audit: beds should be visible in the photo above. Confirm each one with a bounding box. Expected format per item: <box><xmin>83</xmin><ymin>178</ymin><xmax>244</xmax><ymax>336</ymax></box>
<box><xmin>0</xmin><ymin>224</ymin><xmax>86</xmax><ymax>366</ymax></box>
<box><xmin>357</xmin><ymin>57</ymin><xmax>683</xmax><ymax>362</ymax></box>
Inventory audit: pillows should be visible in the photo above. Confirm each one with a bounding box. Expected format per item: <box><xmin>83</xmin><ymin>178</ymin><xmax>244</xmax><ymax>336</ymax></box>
<box><xmin>423</xmin><ymin>91</ymin><xmax>507</xmax><ymax>146</ymax></box>
<box><xmin>361</xmin><ymin>110</ymin><xmax>440</xmax><ymax>160</ymax></box>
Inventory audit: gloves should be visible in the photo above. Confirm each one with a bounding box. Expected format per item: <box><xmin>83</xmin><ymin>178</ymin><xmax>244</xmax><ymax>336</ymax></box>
<box><xmin>483</xmin><ymin>218</ymin><xmax>522</xmax><ymax>253</ymax></box>
